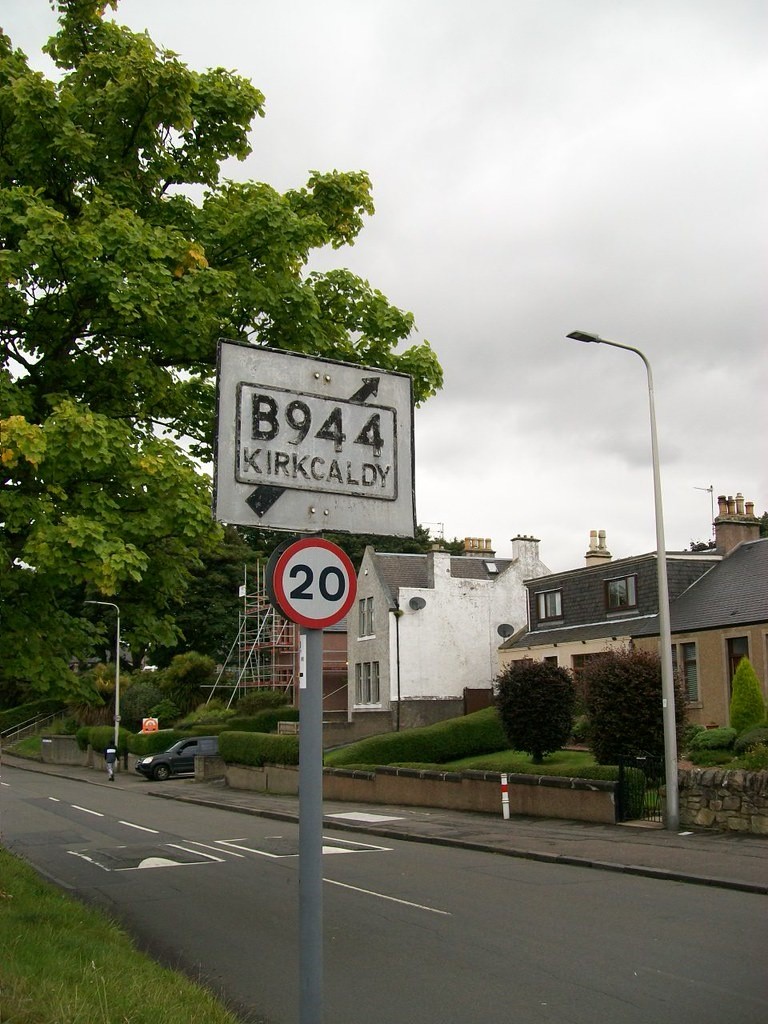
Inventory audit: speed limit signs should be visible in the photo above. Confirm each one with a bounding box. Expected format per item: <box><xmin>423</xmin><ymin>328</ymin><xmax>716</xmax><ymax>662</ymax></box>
<box><xmin>270</xmin><ymin>535</ymin><xmax>359</xmax><ymax>626</ymax></box>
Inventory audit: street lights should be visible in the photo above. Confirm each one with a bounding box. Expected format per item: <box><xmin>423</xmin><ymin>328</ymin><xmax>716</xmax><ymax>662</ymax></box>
<box><xmin>565</xmin><ymin>329</ymin><xmax>681</xmax><ymax>829</ymax></box>
<box><xmin>85</xmin><ymin>600</ymin><xmax>121</xmax><ymax>770</ymax></box>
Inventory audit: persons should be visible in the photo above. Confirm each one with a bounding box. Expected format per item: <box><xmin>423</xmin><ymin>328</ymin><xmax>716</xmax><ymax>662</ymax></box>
<box><xmin>104</xmin><ymin>739</ymin><xmax>120</xmax><ymax>781</ymax></box>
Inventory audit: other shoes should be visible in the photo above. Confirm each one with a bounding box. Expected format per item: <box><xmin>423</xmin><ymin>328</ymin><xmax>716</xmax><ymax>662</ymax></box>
<box><xmin>109</xmin><ymin>778</ymin><xmax>112</xmax><ymax>781</ymax></box>
<box><xmin>111</xmin><ymin>774</ymin><xmax>115</xmax><ymax>781</ymax></box>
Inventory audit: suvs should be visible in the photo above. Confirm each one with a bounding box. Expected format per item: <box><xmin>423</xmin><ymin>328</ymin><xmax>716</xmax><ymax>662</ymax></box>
<box><xmin>135</xmin><ymin>735</ymin><xmax>221</xmax><ymax>781</ymax></box>
